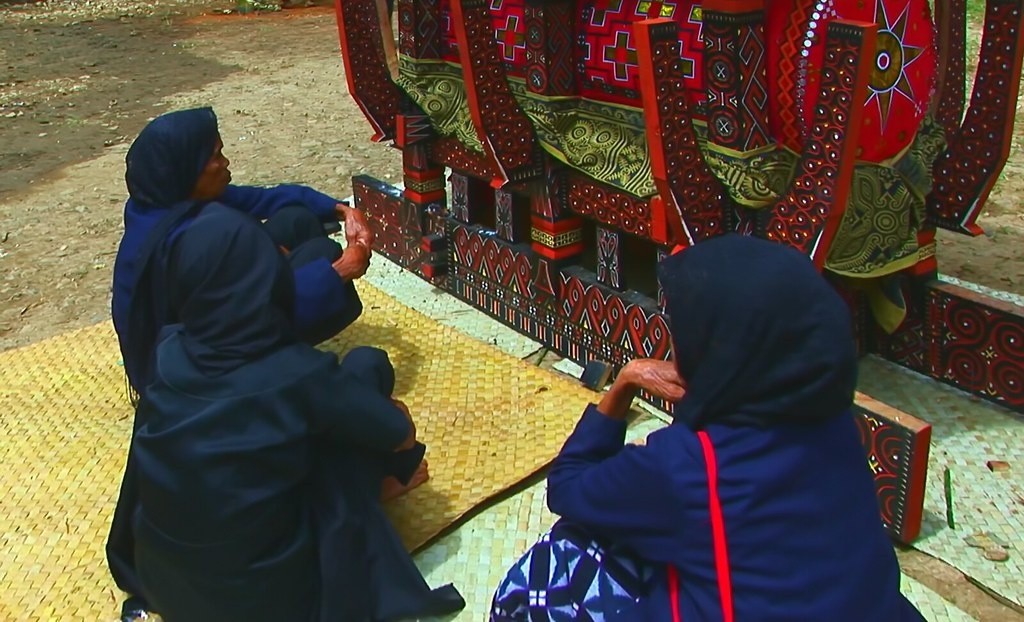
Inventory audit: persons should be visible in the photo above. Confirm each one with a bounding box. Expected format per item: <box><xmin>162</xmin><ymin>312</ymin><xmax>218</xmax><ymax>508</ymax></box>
<box><xmin>104</xmin><ymin>200</ymin><xmax>467</xmax><ymax>622</ymax></box>
<box><xmin>111</xmin><ymin>105</ymin><xmax>376</xmax><ymax>413</ymax></box>
<box><xmin>488</xmin><ymin>233</ymin><xmax>928</xmax><ymax>622</ymax></box>
